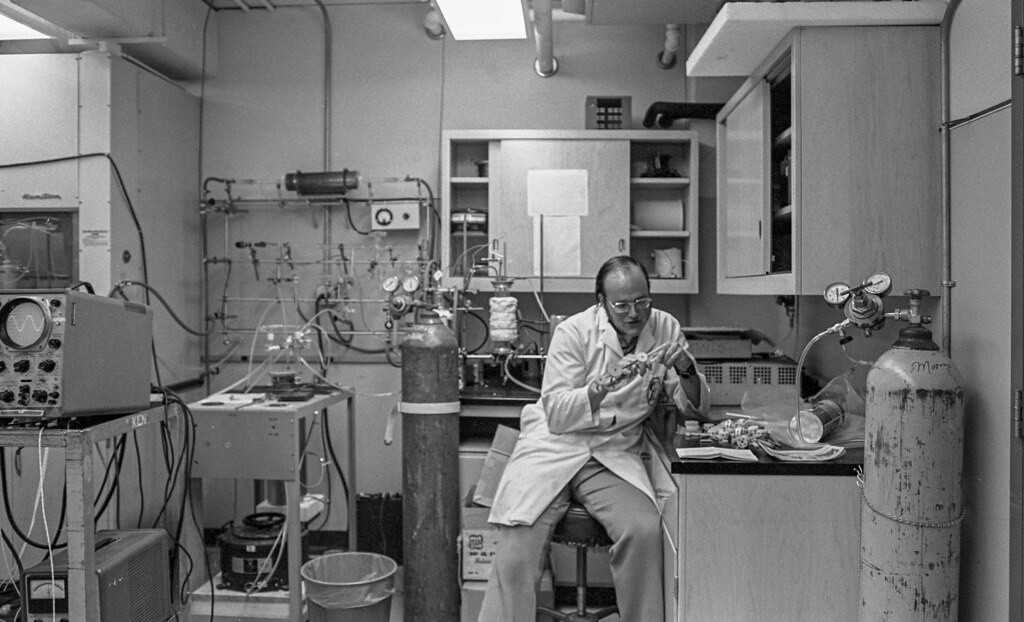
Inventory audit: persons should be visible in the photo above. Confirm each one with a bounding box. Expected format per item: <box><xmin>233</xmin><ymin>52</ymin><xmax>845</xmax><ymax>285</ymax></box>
<box><xmin>476</xmin><ymin>254</ymin><xmax>713</xmax><ymax>622</ymax></box>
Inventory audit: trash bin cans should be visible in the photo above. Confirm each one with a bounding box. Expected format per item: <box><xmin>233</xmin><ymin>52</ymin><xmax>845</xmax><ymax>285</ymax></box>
<box><xmin>299</xmin><ymin>552</ymin><xmax>398</xmax><ymax>622</ymax></box>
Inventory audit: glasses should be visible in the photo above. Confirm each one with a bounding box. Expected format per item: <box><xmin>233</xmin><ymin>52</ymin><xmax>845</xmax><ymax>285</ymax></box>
<box><xmin>604</xmin><ymin>297</ymin><xmax>652</xmax><ymax>313</ymax></box>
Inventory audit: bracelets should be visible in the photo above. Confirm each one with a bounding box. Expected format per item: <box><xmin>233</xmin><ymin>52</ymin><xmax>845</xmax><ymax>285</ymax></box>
<box><xmin>672</xmin><ymin>362</ymin><xmax>696</xmax><ymax>379</ymax></box>
